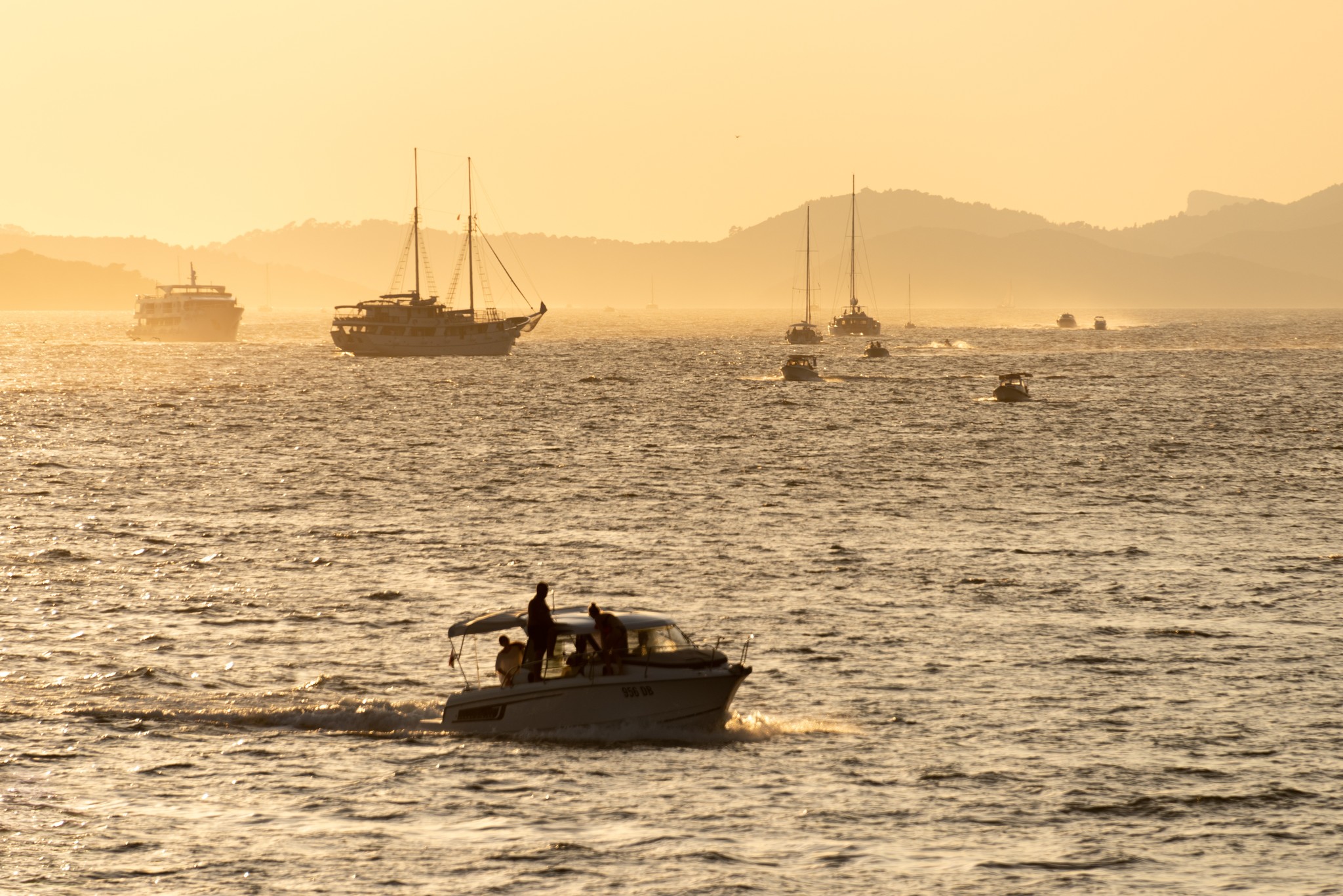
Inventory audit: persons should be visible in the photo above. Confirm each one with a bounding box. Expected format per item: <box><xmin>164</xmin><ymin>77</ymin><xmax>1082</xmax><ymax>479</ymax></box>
<box><xmin>876</xmin><ymin>341</ymin><xmax>881</xmax><ymax>348</ymax></box>
<box><xmin>589</xmin><ymin>602</ymin><xmax>629</xmax><ymax>675</ymax></box>
<box><xmin>870</xmin><ymin>341</ymin><xmax>874</xmax><ymax>348</ymax></box>
<box><xmin>495</xmin><ymin>635</ymin><xmax>526</xmax><ymax>686</ymax></box>
<box><xmin>526</xmin><ymin>581</ymin><xmax>557</xmax><ymax>682</ymax></box>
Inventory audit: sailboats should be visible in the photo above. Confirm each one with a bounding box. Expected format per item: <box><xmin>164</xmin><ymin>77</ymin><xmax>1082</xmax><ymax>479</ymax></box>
<box><xmin>904</xmin><ymin>274</ymin><xmax>916</xmax><ymax>329</ymax></box>
<box><xmin>784</xmin><ymin>205</ymin><xmax>823</xmax><ymax>345</ymax></box>
<box><xmin>827</xmin><ymin>174</ymin><xmax>881</xmax><ymax>336</ymax></box>
<box><xmin>331</xmin><ymin>148</ymin><xmax>548</xmax><ymax>356</ymax></box>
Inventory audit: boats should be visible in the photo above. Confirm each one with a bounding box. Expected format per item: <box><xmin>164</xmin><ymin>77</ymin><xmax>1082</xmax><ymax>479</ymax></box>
<box><xmin>992</xmin><ymin>372</ymin><xmax>1033</xmax><ymax>401</ymax></box>
<box><xmin>943</xmin><ymin>338</ymin><xmax>952</xmax><ymax>346</ymax></box>
<box><xmin>126</xmin><ymin>261</ymin><xmax>245</xmax><ymax>340</ymax></box>
<box><xmin>864</xmin><ymin>339</ymin><xmax>889</xmax><ymax>357</ymax></box>
<box><xmin>1056</xmin><ymin>313</ymin><xmax>1076</xmax><ymax>328</ymax></box>
<box><xmin>421</xmin><ymin>590</ymin><xmax>753</xmax><ymax>731</ymax></box>
<box><xmin>1094</xmin><ymin>316</ymin><xmax>1106</xmax><ymax>330</ymax></box>
<box><xmin>780</xmin><ymin>354</ymin><xmax>819</xmax><ymax>380</ymax></box>
